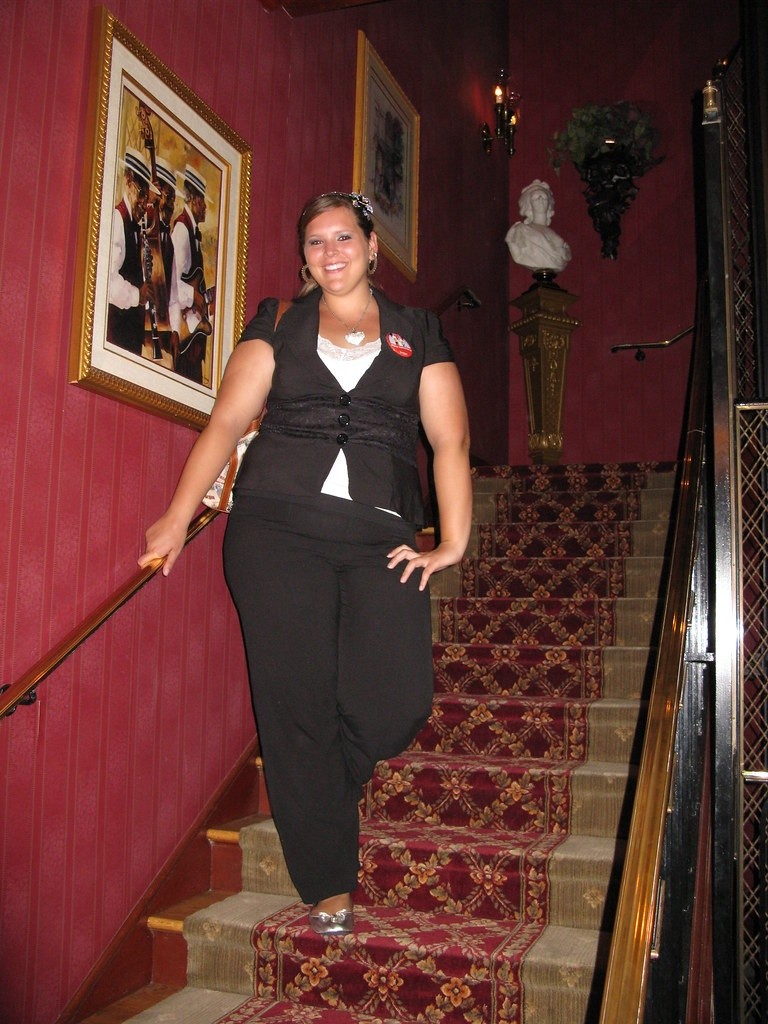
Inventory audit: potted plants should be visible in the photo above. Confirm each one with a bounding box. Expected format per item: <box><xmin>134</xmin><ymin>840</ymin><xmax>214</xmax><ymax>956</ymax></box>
<box><xmin>545</xmin><ymin>95</ymin><xmax>671</xmax><ymax>261</ymax></box>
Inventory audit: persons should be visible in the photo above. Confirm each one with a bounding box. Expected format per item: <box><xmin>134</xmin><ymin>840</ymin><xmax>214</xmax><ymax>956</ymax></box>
<box><xmin>142</xmin><ymin>155</ymin><xmax>181</xmax><ymax>373</ymax></box>
<box><xmin>170</xmin><ymin>163</ymin><xmax>208</xmax><ymax>385</ymax></box>
<box><xmin>504</xmin><ymin>179</ymin><xmax>571</xmax><ymax>273</ymax></box>
<box><xmin>108</xmin><ymin>146</ymin><xmax>154</xmax><ymax>357</ymax></box>
<box><xmin>372</xmin><ymin>108</ymin><xmax>404</xmax><ymax>218</ymax></box>
<box><xmin>138</xmin><ymin>192</ymin><xmax>474</xmax><ymax>934</ymax></box>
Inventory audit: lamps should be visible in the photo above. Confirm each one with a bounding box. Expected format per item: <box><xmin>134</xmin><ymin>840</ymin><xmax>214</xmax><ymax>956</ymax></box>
<box><xmin>478</xmin><ymin>67</ymin><xmax>520</xmax><ymax>156</ymax></box>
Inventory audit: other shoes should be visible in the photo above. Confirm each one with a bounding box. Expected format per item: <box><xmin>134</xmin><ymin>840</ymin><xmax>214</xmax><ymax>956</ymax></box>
<box><xmin>307</xmin><ymin>894</ymin><xmax>357</xmax><ymax>935</ymax></box>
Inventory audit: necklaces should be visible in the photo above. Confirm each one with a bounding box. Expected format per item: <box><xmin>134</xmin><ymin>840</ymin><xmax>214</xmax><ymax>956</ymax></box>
<box><xmin>320</xmin><ymin>287</ymin><xmax>373</xmax><ymax>346</ymax></box>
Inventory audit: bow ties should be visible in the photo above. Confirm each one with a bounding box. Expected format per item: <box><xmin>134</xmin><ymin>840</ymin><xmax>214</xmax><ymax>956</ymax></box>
<box><xmin>191</xmin><ymin>230</ymin><xmax>203</xmax><ymax>242</ymax></box>
<box><xmin>157</xmin><ymin>221</ymin><xmax>171</xmax><ymax>233</ymax></box>
<box><xmin>130</xmin><ymin>220</ymin><xmax>143</xmax><ymax>234</ymax></box>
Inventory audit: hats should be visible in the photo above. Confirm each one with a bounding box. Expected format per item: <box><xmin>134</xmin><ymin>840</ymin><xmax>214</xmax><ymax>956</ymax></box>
<box><xmin>174</xmin><ymin>164</ymin><xmax>215</xmax><ymax>204</ymax></box>
<box><xmin>149</xmin><ymin>154</ymin><xmax>186</xmax><ymax>201</ymax></box>
<box><xmin>118</xmin><ymin>145</ymin><xmax>162</xmax><ymax>194</ymax></box>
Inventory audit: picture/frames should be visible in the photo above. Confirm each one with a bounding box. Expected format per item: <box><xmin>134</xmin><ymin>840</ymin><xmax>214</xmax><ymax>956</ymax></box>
<box><xmin>350</xmin><ymin>30</ymin><xmax>422</xmax><ymax>284</ymax></box>
<box><xmin>68</xmin><ymin>1</ymin><xmax>253</xmax><ymax>434</ymax></box>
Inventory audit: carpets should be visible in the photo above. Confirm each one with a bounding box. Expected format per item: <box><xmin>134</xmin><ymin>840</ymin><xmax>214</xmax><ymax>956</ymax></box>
<box><xmin>116</xmin><ymin>455</ymin><xmax>678</xmax><ymax>1024</ymax></box>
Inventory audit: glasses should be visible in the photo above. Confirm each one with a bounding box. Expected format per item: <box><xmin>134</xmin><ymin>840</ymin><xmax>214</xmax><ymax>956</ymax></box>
<box><xmin>160</xmin><ymin>191</ymin><xmax>178</xmax><ymax>204</ymax></box>
<box><xmin>129</xmin><ymin>179</ymin><xmax>150</xmax><ymax>202</ymax></box>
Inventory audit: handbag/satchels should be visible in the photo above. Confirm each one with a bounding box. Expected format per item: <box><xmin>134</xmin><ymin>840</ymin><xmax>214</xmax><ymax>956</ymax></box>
<box><xmin>202</xmin><ymin>414</ymin><xmax>265</xmax><ymax>514</ymax></box>
